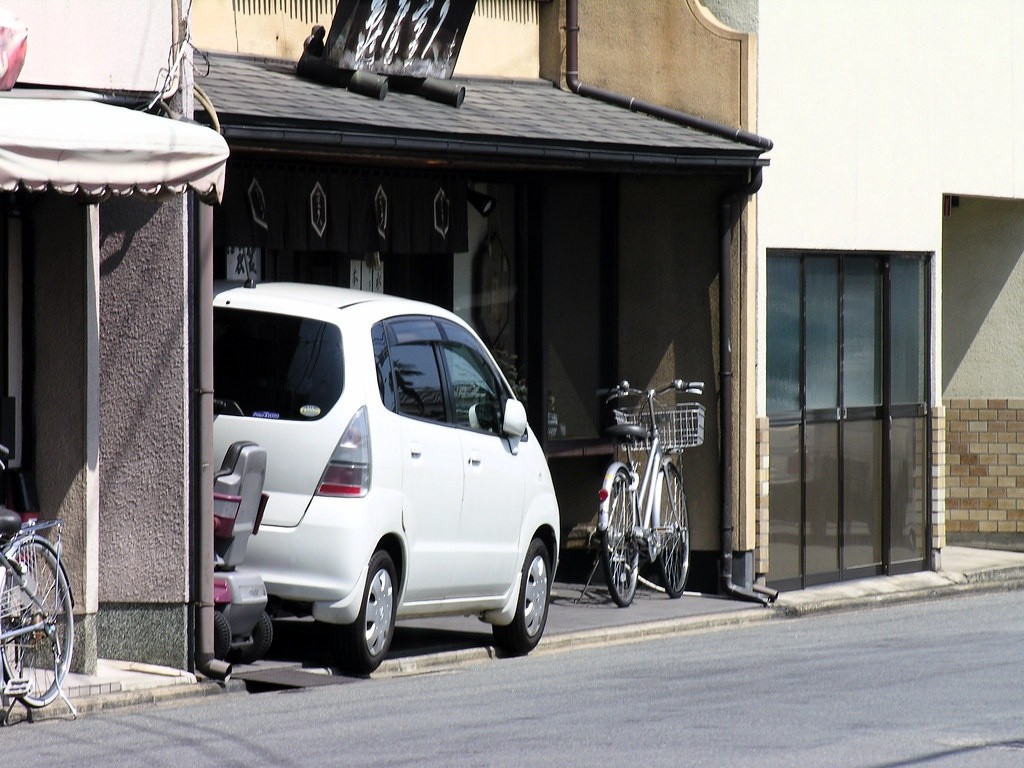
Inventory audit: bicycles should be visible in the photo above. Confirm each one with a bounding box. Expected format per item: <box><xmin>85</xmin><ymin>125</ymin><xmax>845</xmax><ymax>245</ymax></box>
<box><xmin>0</xmin><ymin>509</ymin><xmax>78</xmax><ymax>723</ymax></box>
<box><xmin>598</xmin><ymin>379</ymin><xmax>707</xmax><ymax>609</ymax></box>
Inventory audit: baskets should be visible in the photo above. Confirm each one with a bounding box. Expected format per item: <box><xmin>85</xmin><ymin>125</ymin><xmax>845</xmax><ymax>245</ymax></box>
<box><xmin>613</xmin><ymin>403</ymin><xmax>707</xmax><ymax>450</ymax></box>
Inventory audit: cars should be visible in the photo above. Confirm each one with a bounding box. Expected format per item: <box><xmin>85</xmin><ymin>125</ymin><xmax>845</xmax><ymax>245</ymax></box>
<box><xmin>211</xmin><ymin>281</ymin><xmax>563</xmax><ymax>674</ymax></box>
